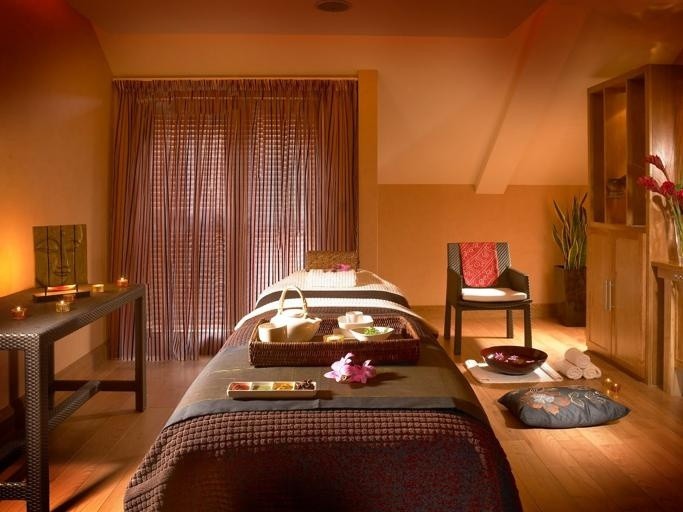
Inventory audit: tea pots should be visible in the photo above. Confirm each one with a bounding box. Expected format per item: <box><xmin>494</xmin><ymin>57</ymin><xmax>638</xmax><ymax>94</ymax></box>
<box><xmin>269</xmin><ymin>286</ymin><xmax>323</xmax><ymax>343</ymax></box>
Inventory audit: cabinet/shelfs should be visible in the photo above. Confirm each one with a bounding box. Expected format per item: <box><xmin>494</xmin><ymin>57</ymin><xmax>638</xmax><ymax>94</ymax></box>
<box><xmin>585</xmin><ymin>64</ymin><xmax>683</xmax><ymax>385</ymax></box>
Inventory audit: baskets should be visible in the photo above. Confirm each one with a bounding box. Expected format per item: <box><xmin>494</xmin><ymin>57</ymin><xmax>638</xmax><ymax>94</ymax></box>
<box><xmin>249</xmin><ymin>308</ymin><xmax>421</xmax><ymax>368</ymax></box>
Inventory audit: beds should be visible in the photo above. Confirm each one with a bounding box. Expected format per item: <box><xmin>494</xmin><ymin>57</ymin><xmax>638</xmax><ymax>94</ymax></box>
<box><xmin>125</xmin><ymin>268</ymin><xmax>525</xmax><ymax>512</ymax></box>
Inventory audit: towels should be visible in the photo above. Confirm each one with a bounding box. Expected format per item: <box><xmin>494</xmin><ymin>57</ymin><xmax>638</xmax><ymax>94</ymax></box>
<box><xmin>564</xmin><ymin>347</ymin><xmax>591</xmax><ymax>368</ymax></box>
<box><xmin>555</xmin><ymin>359</ymin><xmax>583</xmax><ymax>379</ymax></box>
<box><xmin>464</xmin><ymin>359</ymin><xmax>564</xmax><ymax>383</ymax></box>
<box><xmin>583</xmin><ymin>361</ymin><xmax>601</xmax><ymax>379</ymax></box>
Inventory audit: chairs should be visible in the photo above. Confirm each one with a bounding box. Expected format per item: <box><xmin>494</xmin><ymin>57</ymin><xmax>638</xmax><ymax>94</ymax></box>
<box><xmin>303</xmin><ymin>250</ymin><xmax>360</xmax><ymax>270</ymax></box>
<box><xmin>444</xmin><ymin>242</ymin><xmax>532</xmax><ymax>354</ymax></box>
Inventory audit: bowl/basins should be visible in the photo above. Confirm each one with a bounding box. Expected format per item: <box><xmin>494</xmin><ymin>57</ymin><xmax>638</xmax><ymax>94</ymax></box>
<box><xmin>336</xmin><ymin>315</ymin><xmax>373</xmax><ymax>334</ymax></box>
<box><xmin>350</xmin><ymin>326</ymin><xmax>395</xmax><ymax>341</ymax></box>
<box><xmin>480</xmin><ymin>345</ymin><xmax>547</xmax><ymax>376</ymax></box>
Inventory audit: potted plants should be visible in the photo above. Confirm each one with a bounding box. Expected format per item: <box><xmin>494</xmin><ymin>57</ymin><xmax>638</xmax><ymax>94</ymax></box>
<box><xmin>551</xmin><ymin>193</ymin><xmax>588</xmax><ymax>327</ymax></box>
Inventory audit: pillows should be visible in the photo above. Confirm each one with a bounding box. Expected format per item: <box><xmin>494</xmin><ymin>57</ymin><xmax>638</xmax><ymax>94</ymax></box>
<box><xmin>498</xmin><ymin>385</ymin><xmax>632</xmax><ymax>429</ymax></box>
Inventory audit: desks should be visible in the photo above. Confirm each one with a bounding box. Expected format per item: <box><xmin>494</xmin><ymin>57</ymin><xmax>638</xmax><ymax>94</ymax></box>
<box><xmin>0</xmin><ymin>281</ymin><xmax>147</xmax><ymax>512</ymax></box>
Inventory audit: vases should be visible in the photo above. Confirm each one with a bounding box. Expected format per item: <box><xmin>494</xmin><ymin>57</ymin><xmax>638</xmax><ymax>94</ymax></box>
<box><xmin>651</xmin><ymin>257</ymin><xmax>683</xmax><ymax>396</ymax></box>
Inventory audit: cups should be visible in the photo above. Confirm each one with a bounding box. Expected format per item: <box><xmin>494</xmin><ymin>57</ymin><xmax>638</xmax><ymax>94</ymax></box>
<box><xmin>93</xmin><ymin>284</ymin><xmax>104</xmax><ymax>293</ymax></box>
<box><xmin>11</xmin><ymin>307</ymin><xmax>29</xmax><ymax>321</ymax></box>
<box><xmin>347</xmin><ymin>311</ymin><xmax>363</xmax><ymax>324</ymax></box>
<box><xmin>56</xmin><ymin>301</ymin><xmax>71</xmax><ymax>314</ymax></box>
<box><xmin>116</xmin><ymin>278</ymin><xmax>128</xmax><ymax>288</ymax></box>
<box><xmin>258</xmin><ymin>321</ymin><xmax>288</xmax><ymax>344</ymax></box>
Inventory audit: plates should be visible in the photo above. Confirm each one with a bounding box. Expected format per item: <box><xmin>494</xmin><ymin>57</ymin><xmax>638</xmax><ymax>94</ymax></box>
<box><xmin>226</xmin><ymin>380</ymin><xmax>316</xmax><ymax>398</ymax></box>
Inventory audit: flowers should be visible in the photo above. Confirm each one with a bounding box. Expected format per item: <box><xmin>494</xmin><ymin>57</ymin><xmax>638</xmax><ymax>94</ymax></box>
<box><xmin>636</xmin><ymin>155</ymin><xmax>683</xmax><ymax>263</ymax></box>
